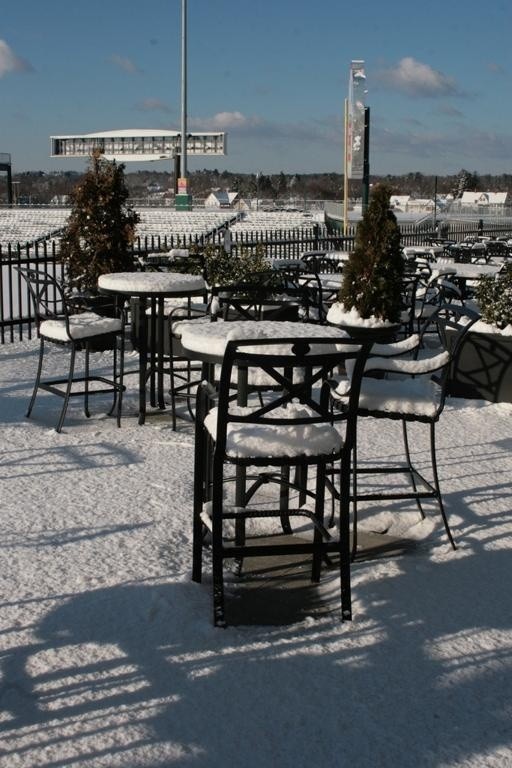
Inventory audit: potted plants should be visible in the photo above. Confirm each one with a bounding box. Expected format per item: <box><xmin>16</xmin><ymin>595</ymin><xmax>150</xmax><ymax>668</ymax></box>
<box><xmin>328</xmin><ymin>181</ymin><xmax>410</xmax><ymax>381</ymax></box>
<box><xmin>59</xmin><ymin>145</ymin><xmax>142</xmax><ymax>352</ymax></box>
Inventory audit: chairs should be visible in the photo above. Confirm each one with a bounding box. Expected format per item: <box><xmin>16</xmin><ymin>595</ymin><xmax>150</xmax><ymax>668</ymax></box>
<box><xmin>1</xmin><ymin>205</ymin><xmax>322</xmax><ymax>261</ymax></box>
<box><xmin>14</xmin><ymin>264</ymin><xmax>132</xmax><ymax>432</ymax></box>
<box><xmin>327</xmin><ymin>297</ymin><xmax>483</xmax><ymax>555</ymax></box>
<box><xmin>189</xmin><ymin>333</ymin><xmax>376</xmax><ymax>632</ymax></box>
<box><xmin>159</xmin><ymin>228</ymin><xmax>511</xmax><ymax>434</ymax></box>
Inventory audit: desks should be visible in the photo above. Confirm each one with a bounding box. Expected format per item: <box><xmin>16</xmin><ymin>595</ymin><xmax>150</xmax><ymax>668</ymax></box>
<box><xmin>93</xmin><ymin>264</ymin><xmax>209</xmax><ymax>429</ymax></box>
<box><xmin>181</xmin><ymin>317</ymin><xmax>352</xmax><ymax>579</ymax></box>
